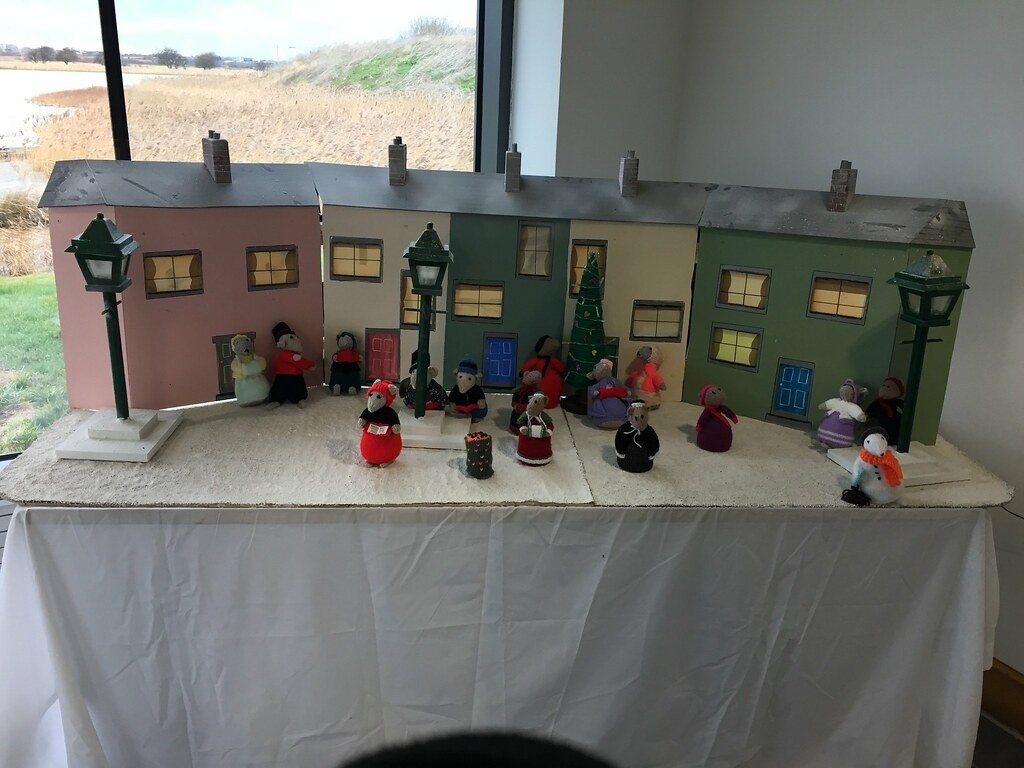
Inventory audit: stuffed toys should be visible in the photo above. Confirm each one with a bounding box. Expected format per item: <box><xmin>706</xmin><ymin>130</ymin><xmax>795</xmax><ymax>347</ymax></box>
<box><xmin>231</xmin><ymin>320</ymin><xmax>906</xmax><ymax>506</ymax></box>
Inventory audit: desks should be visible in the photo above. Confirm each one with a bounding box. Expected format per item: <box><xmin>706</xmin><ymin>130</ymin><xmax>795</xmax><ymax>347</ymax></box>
<box><xmin>0</xmin><ymin>389</ymin><xmax>1016</xmax><ymax>768</ymax></box>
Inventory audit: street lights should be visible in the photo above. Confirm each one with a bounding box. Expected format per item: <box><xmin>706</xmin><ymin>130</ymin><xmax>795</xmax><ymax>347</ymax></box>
<box><xmin>885</xmin><ymin>250</ymin><xmax>972</xmax><ymax>453</ymax></box>
<box><xmin>63</xmin><ymin>211</ymin><xmax>143</xmax><ymax>421</ymax></box>
<box><xmin>401</xmin><ymin>220</ymin><xmax>454</xmax><ymax>420</ymax></box>
